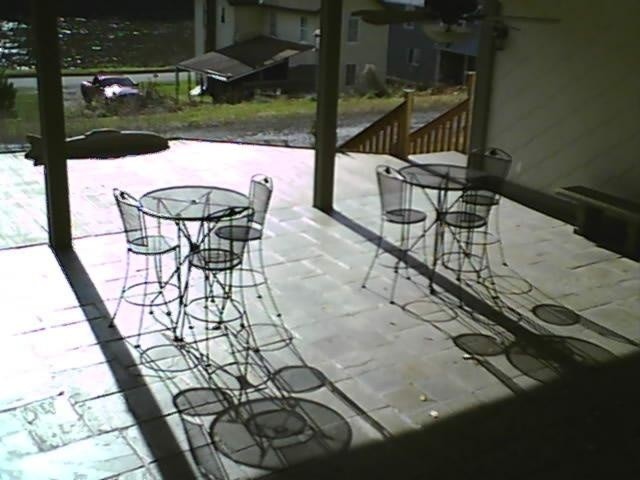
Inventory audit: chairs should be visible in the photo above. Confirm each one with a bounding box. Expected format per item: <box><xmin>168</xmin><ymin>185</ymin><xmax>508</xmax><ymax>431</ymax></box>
<box><xmin>107</xmin><ymin>172</ymin><xmax>282</xmax><ymax>374</ymax></box>
<box><xmin>361</xmin><ymin>143</ymin><xmax>512</xmax><ymax>317</ymax></box>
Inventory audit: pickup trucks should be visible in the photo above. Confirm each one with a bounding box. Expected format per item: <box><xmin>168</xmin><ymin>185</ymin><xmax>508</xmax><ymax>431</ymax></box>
<box><xmin>80</xmin><ymin>71</ymin><xmax>144</xmax><ymax>109</ymax></box>
